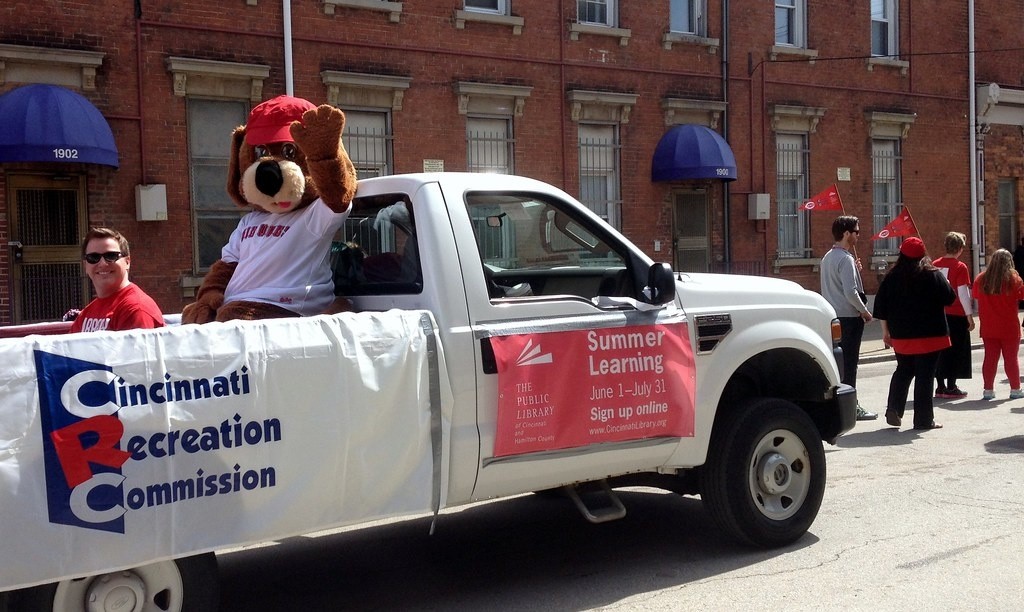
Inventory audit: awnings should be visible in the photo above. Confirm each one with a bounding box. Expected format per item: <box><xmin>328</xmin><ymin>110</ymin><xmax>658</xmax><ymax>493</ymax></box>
<box><xmin>652</xmin><ymin>124</ymin><xmax>738</xmax><ymax>181</ymax></box>
<box><xmin>0</xmin><ymin>84</ymin><xmax>119</xmax><ymax>165</ymax></box>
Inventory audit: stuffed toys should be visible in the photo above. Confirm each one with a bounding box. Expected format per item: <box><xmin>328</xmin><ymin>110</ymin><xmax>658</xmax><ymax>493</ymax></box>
<box><xmin>181</xmin><ymin>95</ymin><xmax>356</xmax><ymax>325</ymax></box>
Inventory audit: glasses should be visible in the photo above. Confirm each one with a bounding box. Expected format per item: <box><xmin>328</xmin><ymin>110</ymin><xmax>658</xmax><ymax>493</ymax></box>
<box><xmin>849</xmin><ymin>229</ymin><xmax>861</xmax><ymax>236</ymax></box>
<box><xmin>83</xmin><ymin>251</ymin><xmax>125</xmax><ymax>265</ymax></box>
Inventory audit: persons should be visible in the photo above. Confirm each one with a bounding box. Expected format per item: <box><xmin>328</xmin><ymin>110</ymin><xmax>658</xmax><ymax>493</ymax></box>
<box><xmin>69</xmin><ymin>227</ymin><xmax>167</xmax><ymax>335</ymax></box>
<box><xmin>932</xmin><ymin>232</ymin><xmax>1024</xmax><ymax>399</ymax></box>
<box><xmin>872</xmin><ymin>236</ymin><xmax>957</xmax><ymax>431</ymax></box>
<box><xmin>821</xmin><ymin>216</ymin><xmax>878</xmax><ymax>420</ymax></box>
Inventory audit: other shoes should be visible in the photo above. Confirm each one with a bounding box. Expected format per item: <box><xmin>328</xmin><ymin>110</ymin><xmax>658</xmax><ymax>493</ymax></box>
<box><xmin>913</xmin><ymin>420</ymin><xmax>943</xmax><ymax>430</ymax></box>
<box><xmin>856</xmin><ymin>404</ymin><xmax>878</xmax><ymax>419</ymax></box>
<box><xmin>885</xmin><ymin>408</ymin><xmax>901</xmax><ymax>426</ymax></box>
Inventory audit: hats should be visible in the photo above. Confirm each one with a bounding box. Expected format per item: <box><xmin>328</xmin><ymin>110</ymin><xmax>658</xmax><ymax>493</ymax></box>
<box><xmin>245</xmin><ymin>95</ymin><xmax>317</xmax><ymax>145</ymax></box>
<box><xmin>899</xmin><ymin>237</ymin><xmax>927</xmax><ymax>258</ymax></box>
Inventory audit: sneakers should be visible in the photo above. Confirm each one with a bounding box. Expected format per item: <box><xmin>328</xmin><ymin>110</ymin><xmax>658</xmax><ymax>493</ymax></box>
<box><xmin>944</xmin><ymin>386</ymin><xmax>968</xmax><ymax>398</ymax></box>
<box><xmin>1010</xmin><ymin>387</ymin><xmax>1024</xmax><ymax>398</ymax></box>
<box><xmin>983</xmin><ymin>389</ymin><xmax>996</xmax><ymax>399</ymax></box>
<box><xmin>935</xmin><ymin>386</ymin><xmax>947</xmax><ymax>398</ymax></box>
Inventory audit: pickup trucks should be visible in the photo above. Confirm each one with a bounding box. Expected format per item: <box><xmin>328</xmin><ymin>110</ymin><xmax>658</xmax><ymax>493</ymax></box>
<box><xmin>0</xmin><ymin>172</ymin><xmax>859</xmax><ymax>612</ymax></box>
